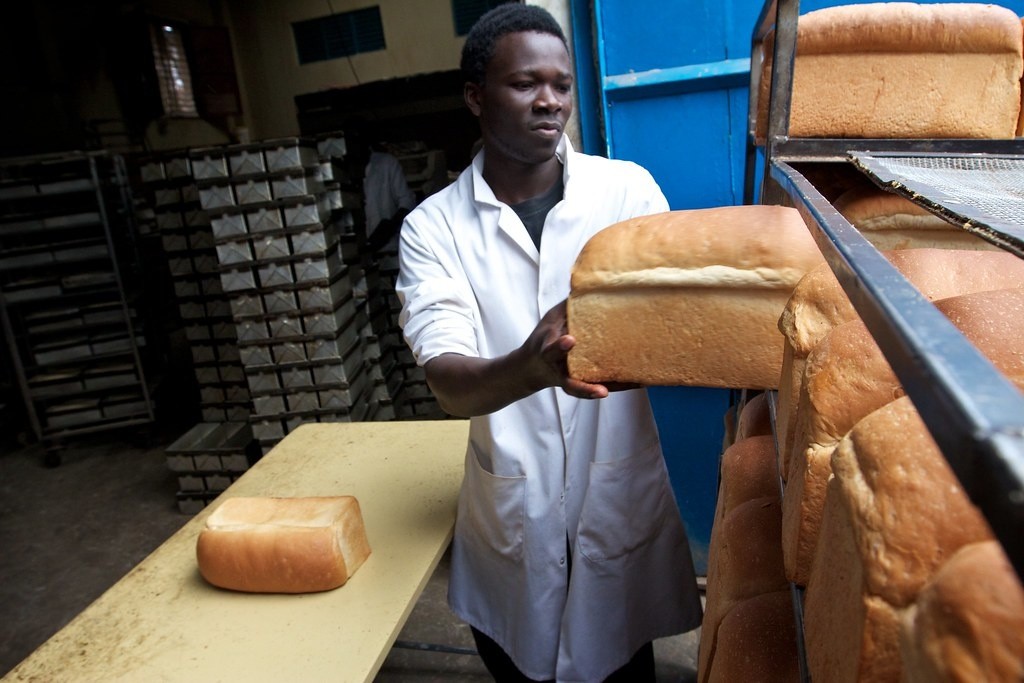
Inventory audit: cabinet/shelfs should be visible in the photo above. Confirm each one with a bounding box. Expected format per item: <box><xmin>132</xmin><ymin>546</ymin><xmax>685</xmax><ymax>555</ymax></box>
<box><xmin>716</xmin><ymin>1</ymin><xmax>1024</xmax><ymax>683</ymax></box>
<box><xmin>0</xmin><ymin>132</ymin><xmax>451</xmax><ymax>466</ymax></box>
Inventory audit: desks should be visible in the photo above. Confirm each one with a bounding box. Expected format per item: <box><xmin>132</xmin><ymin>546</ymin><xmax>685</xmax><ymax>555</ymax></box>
<box><xmin>0</xmin><ymin>418</ymin><xmax>471</xmax><ymax>683</ymax></box>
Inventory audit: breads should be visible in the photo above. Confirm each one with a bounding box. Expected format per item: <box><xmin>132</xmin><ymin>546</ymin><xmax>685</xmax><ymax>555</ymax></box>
<box><xmin>566</xmin><ymin>3</ymin><xmax>1024</xmax><ymax>683</ymax></box>
<box><xmin>196</xmin><ymin>495</ymin><xmax>371</xmax><ymax>593</ymax></box>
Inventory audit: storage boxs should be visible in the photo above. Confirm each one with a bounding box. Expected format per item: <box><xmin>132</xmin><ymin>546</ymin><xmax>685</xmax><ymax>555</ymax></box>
<box><xmin>140</xmin><ymin>137</ymin><xmax>418</xmax><ymax>517</ymax></box>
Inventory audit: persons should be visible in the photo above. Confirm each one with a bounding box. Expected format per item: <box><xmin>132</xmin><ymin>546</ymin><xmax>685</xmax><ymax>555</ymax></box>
<box><xmin>394</xmin><ymin>3</ymin><xmax>704</xmax><ymax>683</ymax></box>
<box><xmin>337</xmin><ymin>116</ymin><xmax>416</xmax><ymax>270</ymax></box>
